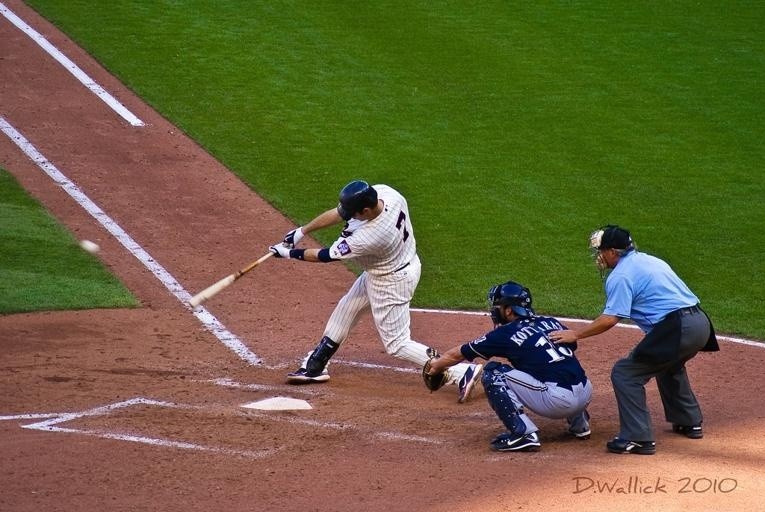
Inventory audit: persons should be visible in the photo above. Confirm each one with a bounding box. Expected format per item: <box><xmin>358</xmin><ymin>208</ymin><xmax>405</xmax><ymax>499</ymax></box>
<box><xmin>269</xmin><ymin>179</ymin><xmax>485</xmax><ymax>404</ymax></box>
<box><xmin>428</xmin><ymin>281</ymin><xmax>593</xmax><ymax>452</ymax></box>
<box><xmin>547</xmin><ymin>225</ymin><xmax>720</xmax><ymax>455</ymax></box>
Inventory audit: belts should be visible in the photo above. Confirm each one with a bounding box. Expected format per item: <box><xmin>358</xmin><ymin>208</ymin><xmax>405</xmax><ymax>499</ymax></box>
<box><xmin>681</xmin><ymin>307</ymin><xmax>698</xmax><ymax>316</ymax></box>
<box><xmin>394</xmin><ymin>262</ymin><xmax>409</xmax><ymax>273</ymax></box>
<box><xmin>556</xmin><ymin>375</ymin><xmax>587</xmax><ymax>391</ymax></box>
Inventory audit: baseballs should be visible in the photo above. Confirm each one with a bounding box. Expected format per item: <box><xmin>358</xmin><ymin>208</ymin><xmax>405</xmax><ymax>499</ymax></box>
<box><xmin>80</xmin><ymin>239</ymin><xmax>99</xmax><ymax>252</ymax></box>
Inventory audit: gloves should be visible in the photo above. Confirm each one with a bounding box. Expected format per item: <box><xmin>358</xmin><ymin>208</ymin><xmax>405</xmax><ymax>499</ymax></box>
<box><xmin>269</xmin><ymin>244</ymin><xmax>294</xmax><ymax>259</ymax></box>
<box><xmin>282</xmin><ymin>227</ymin><xmax>305</xmax><ymax>249</ymax></box>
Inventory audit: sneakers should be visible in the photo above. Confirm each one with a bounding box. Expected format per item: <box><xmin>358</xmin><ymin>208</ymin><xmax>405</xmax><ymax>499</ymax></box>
<box><xmin>673</xmin><ymin>425</ymin><xmax>703</xmax><ymax>438</ymax></box>
<box><xmin>494</xmin><ymin>432</ymin><xmax>541</xmax><ymax>452</ymax></box>
<box><xmin>287</xmin><ymin>368</ymin><xmax>330</xmax><ymax>382</ymax></box>
<box><xmin>568</xmin><ymin>430</ymin><xmax>591</xmax><ymax>441</ymax></box>
<box><xmin>607</xmin><ymin>440</ymin><xmax>656</xmax><ymax>455</ymax></box>
<box><xmin>458</xmin><ymin>364</ymin><xmax>483</xmax><ymax>404</ymax></box>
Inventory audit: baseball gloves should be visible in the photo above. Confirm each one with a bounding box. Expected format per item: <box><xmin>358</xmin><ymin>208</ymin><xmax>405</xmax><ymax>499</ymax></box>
<box><xmin>422</xmin><ymin>347</ymin><xmax>452</xmax><ymax>391</ymax></box>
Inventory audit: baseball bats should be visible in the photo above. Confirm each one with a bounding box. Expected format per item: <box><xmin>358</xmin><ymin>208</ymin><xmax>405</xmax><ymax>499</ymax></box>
<box><xmin>188</xmin><ymin>252</ymin><xmax>275</xmax><ymax>309</ymax></box>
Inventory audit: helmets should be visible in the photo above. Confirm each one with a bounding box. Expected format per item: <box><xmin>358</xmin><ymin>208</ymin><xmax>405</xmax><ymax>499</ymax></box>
<box><xmin>337</xmin><ymin>180</ymin><xmax>377</xmax><ymax>220</ymax></box>
<box><xmin>587</xmin><ymin>225</ymin><xmax>632</xmax><ymax>279</ymax></box>
<box><xmin>487</xmin><ymin>281</ymin><xmax>535</xmax><ymax>330</ymax></box>
